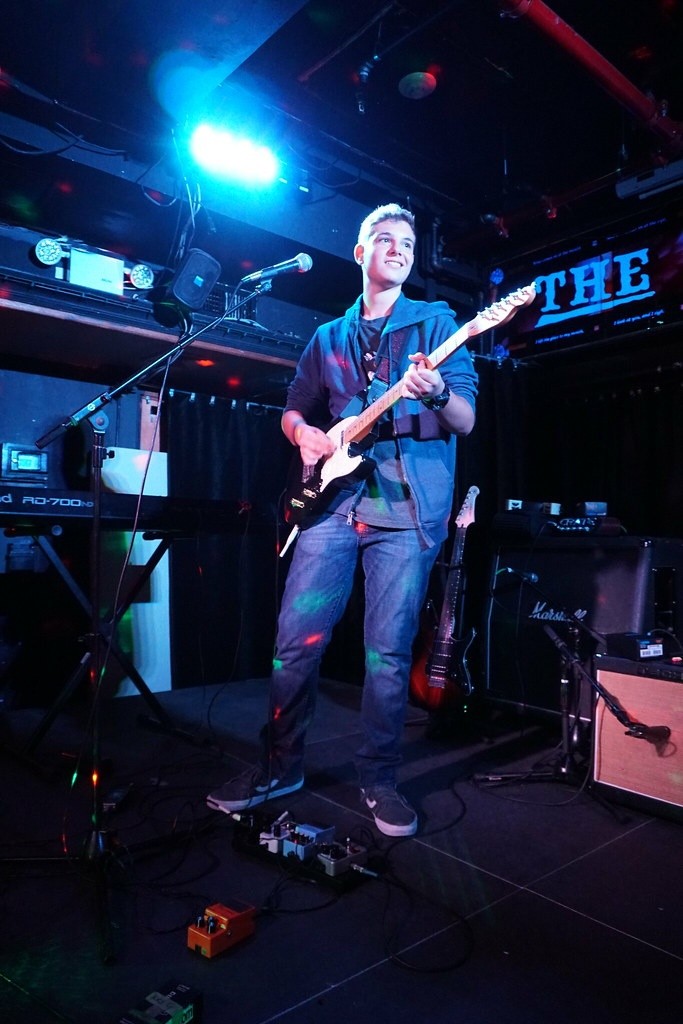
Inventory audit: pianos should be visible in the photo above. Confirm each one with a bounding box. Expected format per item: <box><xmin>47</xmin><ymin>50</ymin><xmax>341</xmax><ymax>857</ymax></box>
<box><xmin>0</xmin><ymin>485</ymin><xmax>278</xmax><ymax>536</ymax></box>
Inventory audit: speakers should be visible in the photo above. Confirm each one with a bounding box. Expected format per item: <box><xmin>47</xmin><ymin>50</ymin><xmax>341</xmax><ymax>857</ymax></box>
<box><xmin>592</xmin><ymin>651</ymin><xmax>683</xmax><ymax>809</ymax></box>
<box><xmin>484</xmin><ymin>535</ymin><xmax>683</xmax><ymax>733</ymax></box>
<box><xmin>165</xmin><ymin>247</ymin><xmax>222</xmax><ymax>313</ymax></box>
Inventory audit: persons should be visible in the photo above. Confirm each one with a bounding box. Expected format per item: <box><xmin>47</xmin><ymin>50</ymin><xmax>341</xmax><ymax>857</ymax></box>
<box><xmin>204</xmin><ymin>203</ymin><xmax>480</xmax><ymax>837</ymax></box>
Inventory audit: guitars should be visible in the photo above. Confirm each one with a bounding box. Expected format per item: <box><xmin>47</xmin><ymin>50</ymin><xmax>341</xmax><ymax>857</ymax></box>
<box><xmin>283</xmin><ymin>285</ymin><xmax>537</xmax><ymax>530</ymax></box>
<box><xmin>410</xmin><ymin>485</ymin><xmax>480</xmax><ymax>709</ymax></box>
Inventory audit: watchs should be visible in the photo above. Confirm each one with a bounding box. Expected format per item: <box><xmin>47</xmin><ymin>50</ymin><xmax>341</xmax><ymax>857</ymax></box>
<box><xmin>421</xmin><ymin>382</ymin><xmax>450</xmax><ymax>411</ymax></box>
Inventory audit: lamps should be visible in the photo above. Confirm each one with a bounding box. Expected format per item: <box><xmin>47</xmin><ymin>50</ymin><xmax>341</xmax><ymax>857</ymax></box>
<box><xmin>34</xmin><ymin>238</ymin><xmax>156</xmax><ymax>296</ymax></box>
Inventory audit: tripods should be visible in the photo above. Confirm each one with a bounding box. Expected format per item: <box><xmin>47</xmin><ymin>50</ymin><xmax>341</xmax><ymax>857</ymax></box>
<box><xmin>0</xmin><ymin>280</ymin><xmax>275</xmax><ymax>972</ymax></box>
<box><xmin>472</xmin><ymin>622</ymin><xmax>632</xmax><ymax>824</ymax></box>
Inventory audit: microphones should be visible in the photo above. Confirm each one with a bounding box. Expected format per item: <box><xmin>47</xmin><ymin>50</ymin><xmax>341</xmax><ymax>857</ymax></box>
<box><xmin>510</xmin><ymin>567</ymin><xmax>538</xmax><ymax>584</ymax></box>
<box><xmin>241</xmin><ymin>251</ymin><xmax>313</xmax><ymax>281</ymax></box>
<box><xmin>626</xmin><ymin>725</ymin><xmax>671</xmax><ymax>743</ymax></box>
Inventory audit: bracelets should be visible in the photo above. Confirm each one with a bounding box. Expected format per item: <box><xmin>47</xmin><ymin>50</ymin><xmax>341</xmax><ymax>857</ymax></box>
<box><xmin>292</xmin><ymin>420</ymin><xmax>306</xmax><ymax>446</ymax></box>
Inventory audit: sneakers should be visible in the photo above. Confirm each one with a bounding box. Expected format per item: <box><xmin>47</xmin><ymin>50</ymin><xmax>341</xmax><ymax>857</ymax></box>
<box><xmin>362</xmin><ymin>782</ymin><xmax>417</xmax><ymax>836</ymax></box>
<box><xmin>205</xmin><ymin>756</ymin><xmax>305</xmax><ymax>812</ymax></box>
